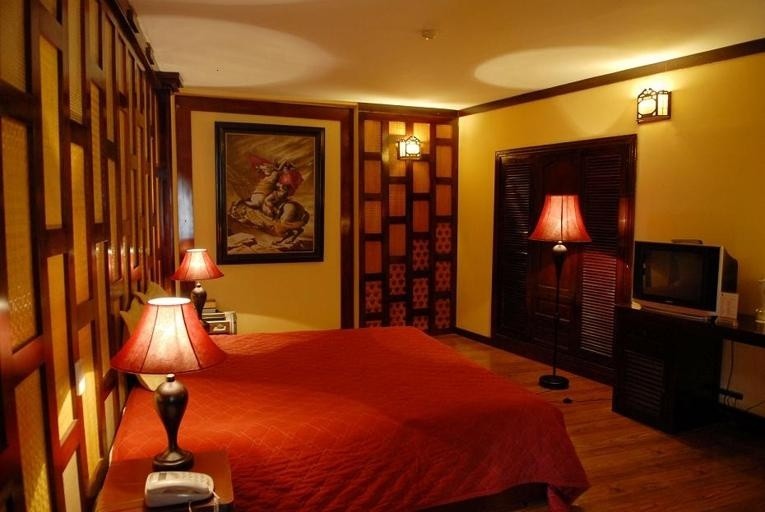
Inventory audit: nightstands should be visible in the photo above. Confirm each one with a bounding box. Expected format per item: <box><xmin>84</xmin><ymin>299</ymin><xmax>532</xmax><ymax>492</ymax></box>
<box><xmin>94</xmin><ymin>449</ymin><xmax>235</xmax><ymax>511</ymax></box>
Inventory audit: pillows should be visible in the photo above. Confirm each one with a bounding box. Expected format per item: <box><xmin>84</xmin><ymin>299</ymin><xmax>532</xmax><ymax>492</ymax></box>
<box><xmin>119</xmin><ymin>281</ymin><xmax>170</xmax><ymax>391</ymax></box>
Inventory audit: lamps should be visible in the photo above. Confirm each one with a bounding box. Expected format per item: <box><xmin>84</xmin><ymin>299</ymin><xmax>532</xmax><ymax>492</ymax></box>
<box><xmin>636</xmin><ymin>87</ymin><xmax>672</xmax><ymax>125</ymax></box>
<box><xmin>395</xmin><ymin>134</ymin><xmax>422</xmax><ymax>161</ymax></box>
<box><xmin>528</xmin><ymin>194</ymin><xmax>593</xmax><ymax>389</ymax></box>
<box><xmin>169</xmin><ymin>248</ymin><xmax>225</xmax><ymax>319</ymax></box>
<box><xmin>107</xmin><ymin>297</ymin><xmax>227</xmax><ymax>468</ymax></box>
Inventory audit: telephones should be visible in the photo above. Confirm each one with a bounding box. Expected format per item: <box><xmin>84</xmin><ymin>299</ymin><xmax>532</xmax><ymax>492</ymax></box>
<box><xmin>144</xmin><ymin>471</ymin><xmax>214</xmax><ymax>508</ymax></box>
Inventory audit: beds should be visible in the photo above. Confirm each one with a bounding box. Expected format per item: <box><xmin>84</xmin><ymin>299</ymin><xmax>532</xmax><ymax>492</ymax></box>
<box><xmin>101</xmin><ymin>325</ymin><xmax>592</xmax><ymax>511</ymax></box>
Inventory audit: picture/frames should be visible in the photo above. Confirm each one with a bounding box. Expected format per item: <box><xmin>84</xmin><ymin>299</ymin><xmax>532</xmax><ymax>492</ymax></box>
<box><xmin>214</xmin><ymin>120</ymin><xmax>325</xmax><ymax>265</ymax></box>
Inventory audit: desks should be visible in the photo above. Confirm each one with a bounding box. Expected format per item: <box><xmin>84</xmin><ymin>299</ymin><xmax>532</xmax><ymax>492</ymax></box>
<box><xmin>611</xmin><ymin>304</ymin><xmax>765</xmax><ymax>434</ymax></box>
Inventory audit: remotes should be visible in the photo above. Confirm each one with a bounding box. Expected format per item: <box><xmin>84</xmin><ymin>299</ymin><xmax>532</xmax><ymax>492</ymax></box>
<box><xmin>673</xmin><ymin>239</ymin><xmax>702</xmax><ymax>244</ymax></box>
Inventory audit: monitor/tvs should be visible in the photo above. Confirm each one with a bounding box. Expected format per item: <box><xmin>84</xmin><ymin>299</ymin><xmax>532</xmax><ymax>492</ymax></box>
<box><xmin>631</xmin><ymin>240</ymin><xmax>738</xmax><ymax>320</ymax></box>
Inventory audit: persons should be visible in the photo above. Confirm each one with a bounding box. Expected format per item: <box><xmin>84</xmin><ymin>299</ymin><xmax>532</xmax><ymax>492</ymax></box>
<box><xmin>264</xmin><ymin>160</ymin><xmax>304</xmax><ymax>216</ymax></box>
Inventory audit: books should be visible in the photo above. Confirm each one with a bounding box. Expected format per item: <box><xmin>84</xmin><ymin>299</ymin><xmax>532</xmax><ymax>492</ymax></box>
<box><xmin>201</xmin><ymin>298</ymin><xmax>225</xmax><ymax>321</ymax></box>
